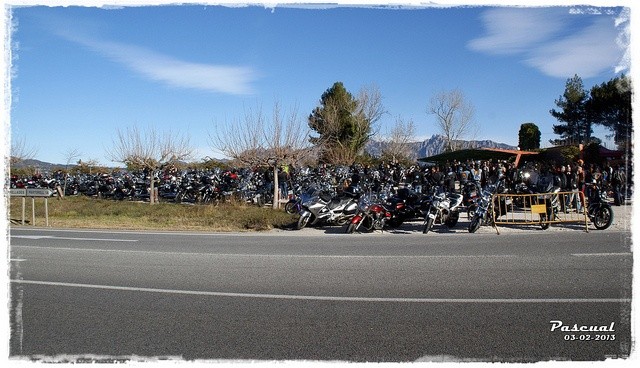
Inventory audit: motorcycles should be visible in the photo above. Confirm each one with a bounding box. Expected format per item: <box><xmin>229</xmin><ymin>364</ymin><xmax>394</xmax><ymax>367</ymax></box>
<box><xmin>584</xmin><ymin>182</ymin><xmax>615</xmax><ymax>230</ymax></box>
<box><xmin>298</xmin><ymin>181</ymin><xmax>365</xmax><ymax>229</ymax></box>
<box><xmin>345</xmin><ymin>174</ymin><xmax>422</xmax><ymax>234</ymax></box>
<box><xmin>534</xmin><ymin>173</ymin><xmax>565</xmax><ymax>230</ymax></box>
<box><xmin>294</xmin><ymin>162</ymin><xmax>541</xmax><ymax>190</ymax></box>
<box><xmin>466</xmin><ymin>175</ymin><xmax>504</xmax><ymax>235</ymax></box>
<box><xmin>10</xmin><ymin>163</ymin><xmax>299</xmax><ymax>214</ymax></box>
<box><xmin>423</xmin><ymin>185</ymin><xmax>468</xmax><ymax>234</ymax></box>
